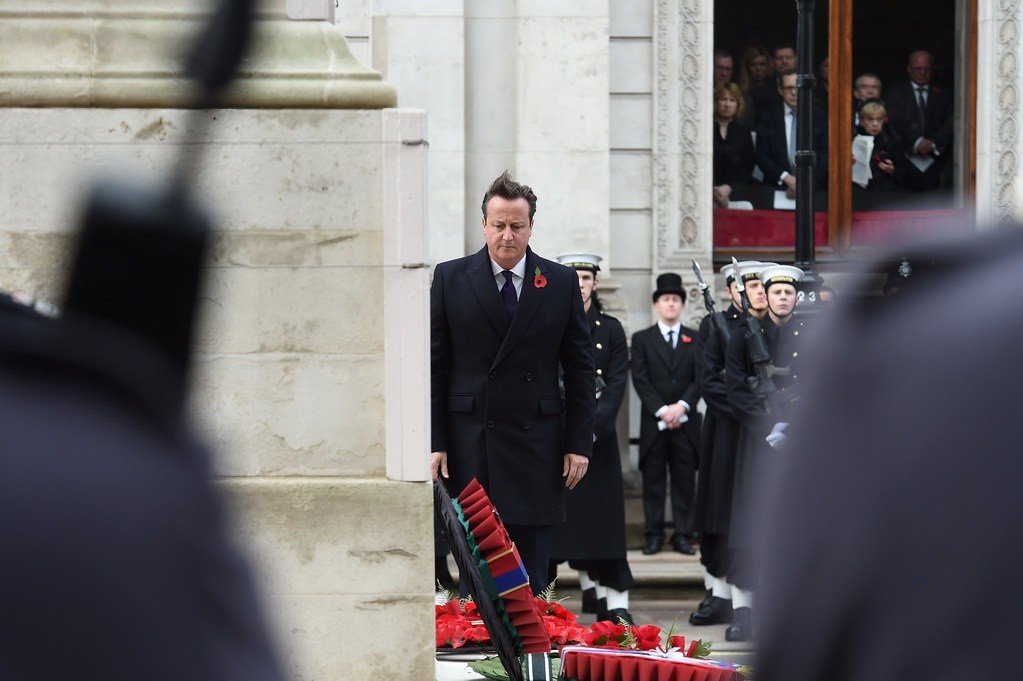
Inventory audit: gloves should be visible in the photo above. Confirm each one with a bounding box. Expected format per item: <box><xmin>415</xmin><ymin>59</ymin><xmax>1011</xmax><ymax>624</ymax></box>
<box><xmin>766</xmin><ymin>432</ymin><xmax>788</xmax><ymax>448</ymax></box>
<box><xmin>770</xmin><ymin>421</ymin><xmax>790</xmax><ymax>432</ymax></box>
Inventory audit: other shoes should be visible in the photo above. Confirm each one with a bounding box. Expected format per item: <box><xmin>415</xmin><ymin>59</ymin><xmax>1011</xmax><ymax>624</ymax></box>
<box><xmin>581</xmin><ymin>587</ymin><xmax>597</xmax><ymax>611</ymax></box>
<box><xmin>595</xmin><ymin>597</ymin><xmax>607</xmax><ymax>621</ymax></box>
<box><xmin>608</xmin><ymin>608</ymin><xmax>635</xmax><ymax>625</ymax></box>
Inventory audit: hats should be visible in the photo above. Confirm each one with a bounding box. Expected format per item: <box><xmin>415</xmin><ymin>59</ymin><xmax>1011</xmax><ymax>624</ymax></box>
<box><xmin>556</xmin><ymin>253</ymin><xmax>603</xmax><ymax>271</ymax></box>
<box><xmin>653</xmin><ymin>272</ymin><xmax>686</xmax><ymax>304</ymax></box>
<box><xmin>761</xmin><ymin>265</ymin><xmax>804</xmax><ymax>294</ymax></box>
<box><xmin>719</xmin><ymin>261</ymin><xmax>758</xmax><ymax>287</ymax></box>
<box><xmin>740</xmin><ymin>261</ymin><xmax>779</xmax><ymax>284</ymax></box>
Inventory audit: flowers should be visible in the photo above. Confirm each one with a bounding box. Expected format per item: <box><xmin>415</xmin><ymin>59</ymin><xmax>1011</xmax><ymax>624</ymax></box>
<box><xmin>534</xmin><ymin>267</ymin><xmax>547</xmax><ymax>288</ymax></box>
<box><xmin>435</xmin><ymin>477</ymin><xmax>754</xmax><ymax>681</ymax></box>
<box><xmin>682</xmin><ymin>335</ymin><xmax>692</xmax><ymax>343</ymax></box>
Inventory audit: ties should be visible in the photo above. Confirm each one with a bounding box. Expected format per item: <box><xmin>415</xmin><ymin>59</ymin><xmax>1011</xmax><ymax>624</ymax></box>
<box><xmin>499</xmin><ymin>271</ymin><xmax>517</xmax><ymax>319</ymax></box>
<box><xmin>668</xmin><ymin>331</ymin><xmax>674</xmax><ymax>349</ymax></box>
<box><xmin>790</xmin><ymin>110</ymin><xmax>796</xmax><ymax>166</ymax></box>
<box><xmin>916</xmin><ymin>87</ymin><xmax>926</xmax><ymax>128</ymax></box>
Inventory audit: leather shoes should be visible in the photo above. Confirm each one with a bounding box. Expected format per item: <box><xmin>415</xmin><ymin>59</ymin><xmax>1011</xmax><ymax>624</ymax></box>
<box><xmin>673</xmin><ymin>536</ymin><xmax>695</xmax><ymax>555</ymax></box>
<box><xmin>726</xmin><ymin>606</ymin><xmax>752</xmax><ymax>641</ymax></box>
<box><xmin>642</xmin><ymin>535</ymin><xmax>664</xmax><ymax>555</ymax></box>
<box><xmin>698</xmin><ymin>586</ymin><xmax>713</xmax><ymax>611</ymax></box>
<box><xmin>689</xmin><ymin>595</ymin><xmax>733</xmax><ymax>625</ymax></box>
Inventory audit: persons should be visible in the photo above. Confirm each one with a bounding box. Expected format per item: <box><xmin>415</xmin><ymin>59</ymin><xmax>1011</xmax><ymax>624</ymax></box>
<box><xmin>434</xmin><ymin>253</ymin><xmax>634</xmax><ymax>626</ymax></box>
<box><xmin>429</xmin><ymin>169</ymin><xmax>593</xmax><ymax>599</ymax></box>
<box><xmin>688</xmin><ymin>260</ymin><xmax>837</xmax><ymax>641</ymax></box>
<box><xmin>629</xmin><ymin>272</ymin><xmax>698</xmax><ymax>555</ymax></box>
<box><xmin>714</xmin><ymin>46</ymin><xmax>955</xmax><ymax>212</ymax></box>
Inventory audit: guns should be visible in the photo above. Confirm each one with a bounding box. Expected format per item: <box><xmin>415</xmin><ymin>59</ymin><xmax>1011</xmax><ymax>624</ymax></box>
<box><xmin>60</xmin><ymin>0</ymin><xmax>268</xmax><ymax>423</ymax></box>
<box><xmin>691</xmin><ymin>257</ymin><xmax>733</xmax><ymax>348</ymax></box>
<box><xmin>730</xmin><ymin>257</ymin><xmax>802</xmax><ymax>415</ymax></box>
